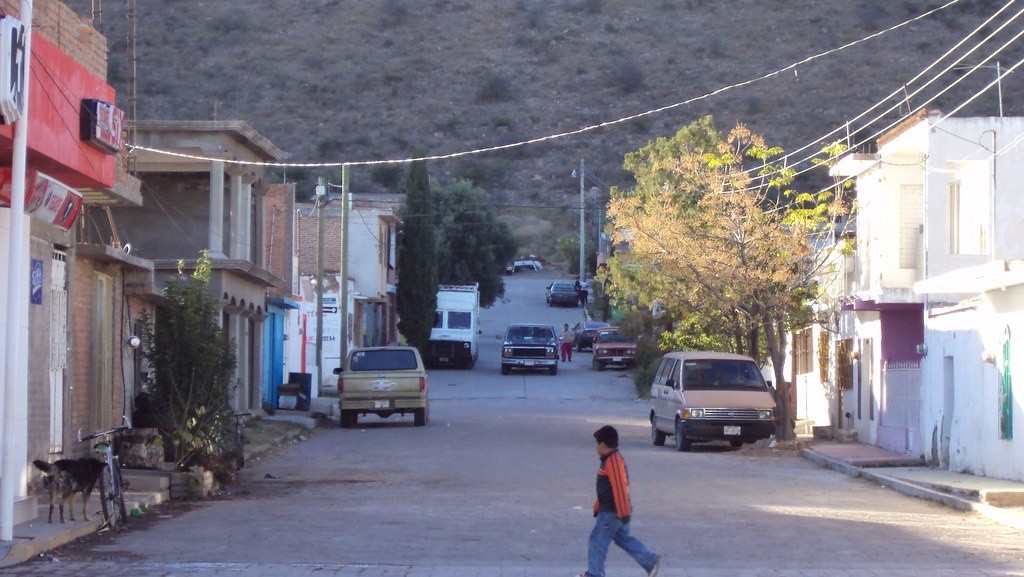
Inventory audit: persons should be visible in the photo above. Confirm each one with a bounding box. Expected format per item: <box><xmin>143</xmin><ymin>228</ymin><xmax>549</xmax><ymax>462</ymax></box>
<box><xmin>558</xmin><ymin>323</ymin><xmax>576</xmax><ymax>362</ymax></box>
<box><xmin>575</xmin><ymin>425</ymin><xmax>662</xmax><ymax>577</ymax></box>
<box><xmin>767</xmin><ymin>380</ymin><xmax>776</xmax><ymax>397</ymax></box>
<box><xmin>580</xmin><ymin>277</ymin><xmax>588</xmax><ymax>304</ymax></box>
<box><xmin>574</xmin><ymin>276</ymin><xmax>585</xmax><ymax>307</ymax></box>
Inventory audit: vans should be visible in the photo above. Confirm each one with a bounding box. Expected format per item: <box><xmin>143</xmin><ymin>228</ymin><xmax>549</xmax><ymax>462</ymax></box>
<box><xmin>430</xmin><ymin>281</ymin><xmax>481</xmax><ymax>369</ymax></box>
<box><xmin>649</xmin><ymin>350</ymin><xmax>775</xmax><ymax>455</ymax></box>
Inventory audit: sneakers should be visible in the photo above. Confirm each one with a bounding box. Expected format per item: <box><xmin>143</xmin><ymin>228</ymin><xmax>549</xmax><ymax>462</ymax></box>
<box><xmin>648</xmin><ymin>556</ymin><xmax>661</xmax><ymax>576</ymax></box>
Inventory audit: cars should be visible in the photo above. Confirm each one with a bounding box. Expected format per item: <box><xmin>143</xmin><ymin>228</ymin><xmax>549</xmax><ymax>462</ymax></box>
<box><xmin>570</xmin><ymin>321</ymin><xmax>612</xmax><ymax>352</ymax></box>
<box><xmin>591</xmin><ymin>327</ymin><xmax>639</xmax><ymax>371</ymax></box>
<box><xmin>545</xmin><ymin>280</ymin><xmax>579</xmax><ymax>308</ymax></box>
<box><xmin>499</xmin><ymin>323</ymin><xmax>559</xmax><ymax>376</ymax></box>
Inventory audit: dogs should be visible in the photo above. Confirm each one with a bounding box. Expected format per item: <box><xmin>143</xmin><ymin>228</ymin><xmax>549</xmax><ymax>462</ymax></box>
<box><xmin>32</xmin><ymin>457</ymin><xmax>111</xmax><ymax>524</ymax></box>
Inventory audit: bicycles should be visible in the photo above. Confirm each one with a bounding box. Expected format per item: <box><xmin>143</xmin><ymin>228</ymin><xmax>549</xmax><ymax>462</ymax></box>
<box><xmin>76</xmin><ymin>415</ymin><xmax>132</xmax><ymax>532</ymax></box>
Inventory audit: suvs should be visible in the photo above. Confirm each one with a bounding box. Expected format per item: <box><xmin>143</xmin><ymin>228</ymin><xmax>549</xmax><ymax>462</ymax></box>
<box><xmin>329</xmin><ymin>343</ymin><xmax>429</xmax><ymax>429</ymax></box>
